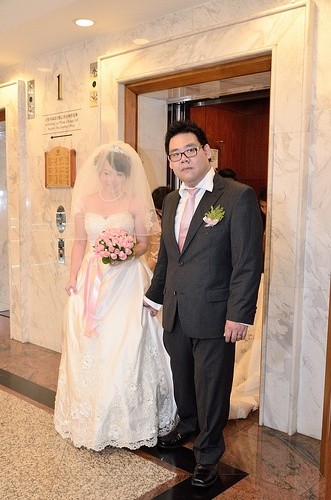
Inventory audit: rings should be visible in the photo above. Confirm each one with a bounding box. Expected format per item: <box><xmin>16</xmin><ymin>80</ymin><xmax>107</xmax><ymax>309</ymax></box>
<box><xmin>237</xmin><ymin>334</ymin><xmax>242</xmax><ymax>336</ymax></box>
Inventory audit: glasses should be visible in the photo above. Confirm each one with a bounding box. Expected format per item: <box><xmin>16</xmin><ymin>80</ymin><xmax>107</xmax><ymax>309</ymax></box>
<box><xmin>167</xmin><ymin>145</ymin><xmax>204</xmax><ymax>162</ymax></box>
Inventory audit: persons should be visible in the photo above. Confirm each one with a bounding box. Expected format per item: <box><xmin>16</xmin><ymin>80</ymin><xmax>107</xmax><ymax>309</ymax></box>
<box><xmin>143</xmin><ymin>121</ymin><xmax>267</xmax><ymax>487</ymax></box>
<box><xmin>53</xmin><ymin>140</ymin><xmax>177</xmax><ymax>452</ymax></box>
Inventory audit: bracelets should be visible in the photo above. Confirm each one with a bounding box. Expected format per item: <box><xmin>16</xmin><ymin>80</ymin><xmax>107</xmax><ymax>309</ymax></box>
<box><xmin>125</xmin><ymin>249</ymin><xmax>135</xmax><ymax>262</ymax></box>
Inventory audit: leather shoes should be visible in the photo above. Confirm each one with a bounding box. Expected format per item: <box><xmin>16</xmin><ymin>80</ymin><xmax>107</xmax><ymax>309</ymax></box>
<box><xmin>192</xmin><ymin>461</ymin><xmax>219</xmax><ymax>487</ymax></box>
<box><xmin>158</xmin><ymin>429</ymin><xmax>189</xmax><ymax>448</ymax></box>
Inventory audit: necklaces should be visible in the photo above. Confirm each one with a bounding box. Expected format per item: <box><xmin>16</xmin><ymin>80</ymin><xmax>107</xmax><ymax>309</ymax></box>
<box><xmin>100</xmin><ymin>189</ymin><xmax>123</xmax><ymax>202</ymax></box>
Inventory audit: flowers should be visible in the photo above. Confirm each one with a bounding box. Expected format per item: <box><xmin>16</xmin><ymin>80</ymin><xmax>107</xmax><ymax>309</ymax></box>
<box><xmin>201</xmin><ymin>206</ymin><xmax>225</xmax><ymax>228</ymax></box>
<box><xmin>93</xmin><ymin>225</ymin><xmax>138</xmax><ymax>263</ymax></box>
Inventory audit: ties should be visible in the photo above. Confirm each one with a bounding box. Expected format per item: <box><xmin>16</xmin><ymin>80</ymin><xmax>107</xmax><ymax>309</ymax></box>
<box><xmin>178</xmin><ymin>187</ymin><xmax>201</xmax><ymax>255</ymax></box>
